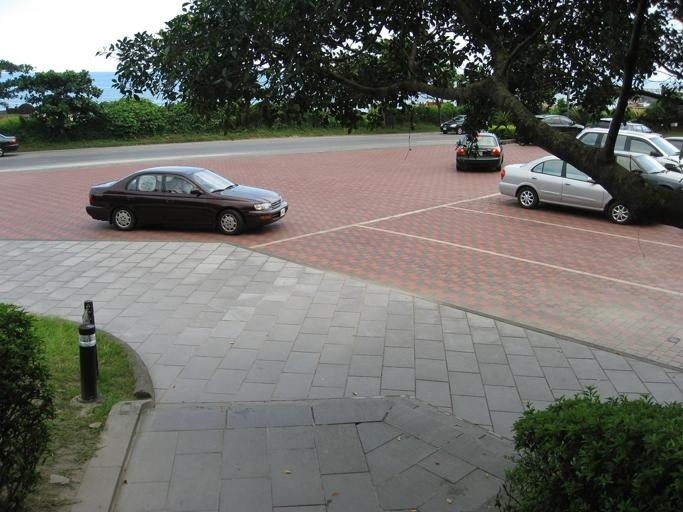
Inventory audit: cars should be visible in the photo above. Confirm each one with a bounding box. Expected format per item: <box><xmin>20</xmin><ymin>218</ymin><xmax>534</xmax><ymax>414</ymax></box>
<box><xmin>440</xmin><ymin>114</ymin><xmax>467</xmax><ymax>135</ymax></box>
<box><xmin>615</xmin><ymin>150</ymin><xmax>683</xmax><ymax>191</ymax></box>
<box><xmin>598</xmin><ymin>118</ymin><xmax>651</xmax><ymax>133</ymax></box>
<box><xmin>85</xmin><ymin>166</ymin><xmax>288</xmax><ymax>235</ymax></box>
<box><xmin>0</xmin><ymin>134</ymin><xmax>18</xmax><ymax>157</ymax></box>
<box><xmin>664</xmin><ymin>136</ymin><xmax>683</xmax><ymax>150</ymax></box>
<box><xmin>455</xmin><ymin>132</ymin><xmax>503</xmax><ymax>172</ymax></box>
<box><xmin>514</xmin><ymin>114</ymin><xmax>584</xmax><ymax>146</ymax></box>
<box><xmin>498</xmin><ymin>155</ymin><xmax>635</xmax><ymax>225</ymax></box>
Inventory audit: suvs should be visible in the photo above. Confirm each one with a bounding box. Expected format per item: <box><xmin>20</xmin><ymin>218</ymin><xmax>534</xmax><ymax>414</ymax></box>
<box><xmin>576</xmin><ymin>127</ymin><xmax>683</xmax><ymax>166</ymax></box>
